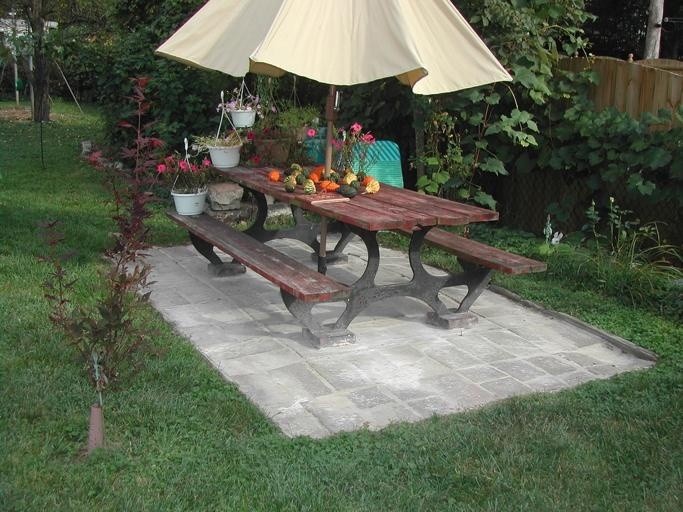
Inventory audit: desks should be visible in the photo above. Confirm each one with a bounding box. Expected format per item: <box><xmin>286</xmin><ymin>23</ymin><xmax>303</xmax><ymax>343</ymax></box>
<box><xmin>210</xmin><ymin>158</ymin><xmax>500</xmax><ymax>349</ymax></box>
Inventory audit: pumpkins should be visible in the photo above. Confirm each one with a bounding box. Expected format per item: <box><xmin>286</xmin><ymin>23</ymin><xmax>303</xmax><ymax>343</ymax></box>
<box><xmin>270</xmin><ymin>163</ymin><xmax>380</xmax><ymax>198</ymax></box>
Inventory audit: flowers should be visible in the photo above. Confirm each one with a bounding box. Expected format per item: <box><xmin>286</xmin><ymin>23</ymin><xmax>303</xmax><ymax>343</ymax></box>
<box><xmin>307</xmin><ymin>121</ymin><xmax>375</xmax><ymax>181</ymax></box>
<box><xmin>190</xmin><ymin>130</ymin><xmax>250</xmax><ymax>152</ymax></box>
<box><xmin>156</xmin><ymin>153</ymin><xmax>213</xmax><ymax>193</ymax></box>
<box><xmin>246</xmin><ymin>126</ymin><xmax>294</xmax><ymax>168</ymax></box>
<box><xmin>216</xmin><ymin>87</ymin><xmax>266</xmax><ymax>120</ymax></box>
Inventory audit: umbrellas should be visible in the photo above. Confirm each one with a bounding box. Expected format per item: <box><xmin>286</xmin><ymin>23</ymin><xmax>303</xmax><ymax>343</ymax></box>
<box><xmin>153</xmin><ymin>0</ymin><xmax>513</xmax><ymax>276</ymax></box>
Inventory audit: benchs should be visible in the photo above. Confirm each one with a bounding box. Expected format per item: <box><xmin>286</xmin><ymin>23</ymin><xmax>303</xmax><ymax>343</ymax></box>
<box><xmin>165</xmin><ymin>211</ymin><xmax>352</xmax><ymax>351</ymax></box>
<box><xmin>399</xmin><ymin>227</ymin><xmax>548</xmax><ymax>331</ymax></box>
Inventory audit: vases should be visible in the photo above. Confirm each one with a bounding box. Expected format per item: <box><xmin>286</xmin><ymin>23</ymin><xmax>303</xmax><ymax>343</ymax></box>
<box><xmin>248</xmin><ymin>137</ymin><xmax>292</xmax><ymax>163</ymax></box>
<box><xmin>171</xmin><ymin>187</ymin><xmax>208</xmax><ymax>217</ymax></box>
<box><xmin>206</xmin><ymin>142</ymin><xmax>243</xmax><ymax>169</ymax></box>
<box><xmin>228</xmin><ymin>109</ymin><xmax>257</xmax><ymax>128</ymax></box>
